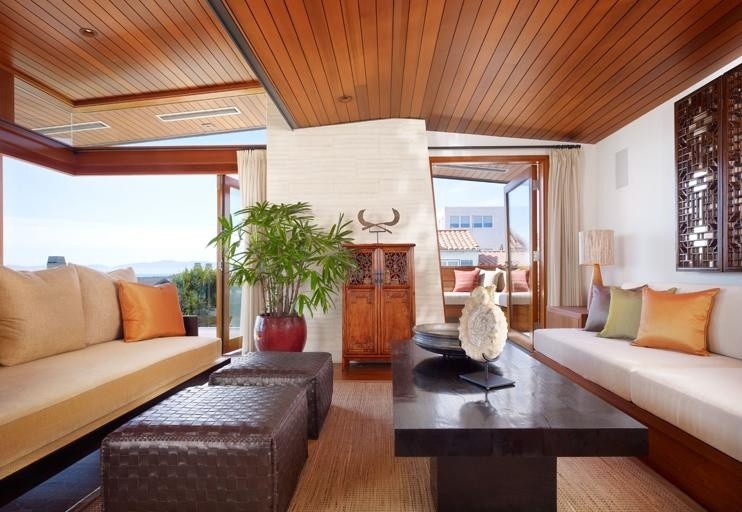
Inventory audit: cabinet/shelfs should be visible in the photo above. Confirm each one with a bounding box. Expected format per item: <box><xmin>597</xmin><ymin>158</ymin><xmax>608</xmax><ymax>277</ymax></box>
<box><xmin>544</xmin><ymin>305</ymin><xmax>588</xmax><ymax>329</ymax></box>
<box><xmin>340</xmin><ymin>243</ymin><xmax>416</xmax><ymax>369</ymax></box>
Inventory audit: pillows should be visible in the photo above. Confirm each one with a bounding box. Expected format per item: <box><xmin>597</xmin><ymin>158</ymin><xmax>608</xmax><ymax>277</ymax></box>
<box><xmin>453</xmin><ymin>269</ymin><xmax>480</xmax><ymax>292</ymax></box>
<box><xmin>502</xmin><ymin>270</ymin><xmax>528</xmax><ymax>292</ymax></box>
<box><xmin>0</xmin><ymin>263</ymin><xmax>86</xmax><ymax>366</ymax></box>
<box><xmin>631</xmin><ymin>288</ymin><xmax>721</xmax><ymax>357</ymax></box>
<box><xmin>598</xmin><ymin>285</ymin><xmax>677</xmax><ymax>339</ymax></box>
<box><xmin>583</xmin><ymin>285</ymin><xmax>644</xmax><ymax>332</ymax></box>
<box><xmin>68</xmin><ymin>263</ymin><xmax>136</xmax><ymax>346</ymax></box>
<box><xmin>497</xmin><ymin>267</ymin><xmax>506</xmax><ymax>291</ymax></box>
<box><xmin>117</xmin><ymin>280</ymin><xmax>187</xmax><ymax>343</ymax></box>
<box><xmin>476</xmin><ymin>268</ymin><xmax>501</xmax><ymax>289</ymax></box>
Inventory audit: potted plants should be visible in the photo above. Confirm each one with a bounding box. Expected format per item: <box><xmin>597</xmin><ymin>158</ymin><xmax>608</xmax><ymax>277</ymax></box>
<box><xmin>205</xmin><ymin>201</ymin><xmax>365</xmax><ymax>352</ymax></box>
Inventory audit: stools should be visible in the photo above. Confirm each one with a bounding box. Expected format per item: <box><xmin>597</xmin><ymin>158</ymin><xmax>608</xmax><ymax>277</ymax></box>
<box><xmin>209</xmin><ymin>351</ymin><xmax>333</xmax><ymax>440</ymax></box>
<box><xmin>100</xmin><ymin>386</ymin><xmax>310</xmax><ymax>511</ymax></box>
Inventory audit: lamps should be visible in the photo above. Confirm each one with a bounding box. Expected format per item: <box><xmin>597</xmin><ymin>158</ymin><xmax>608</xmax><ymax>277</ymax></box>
<box><xmin>578</xmin><ymin>229</ymin><xmax>616</xmax><ymax>312</ymax></box>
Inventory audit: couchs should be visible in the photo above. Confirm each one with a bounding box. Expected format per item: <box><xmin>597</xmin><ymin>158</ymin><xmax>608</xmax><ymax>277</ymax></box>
<box><xmin>534</xmin><ymin>285</ymin><xmax>741</xmax><ymax>511</ymax></box>
<box><xmin>442</xmin><ymin>266</ymin><xmax>530</xmax><ymax>331</ymax></box>
<box><xmin>1</xmin><ymin>262</ymin><xmax>231</xmax><ymax>511</ymax></box>
